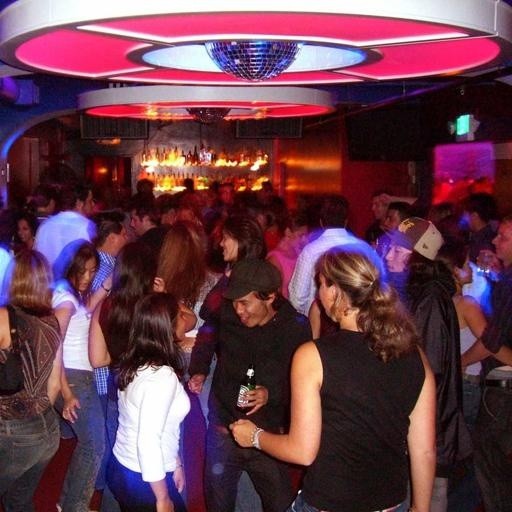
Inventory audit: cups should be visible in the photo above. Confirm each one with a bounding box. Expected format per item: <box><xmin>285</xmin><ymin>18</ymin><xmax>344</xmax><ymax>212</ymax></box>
<box><xmin>476</xmin><ymin>253</ymin><xmax>491</xmax><ymax>277</ymax></box>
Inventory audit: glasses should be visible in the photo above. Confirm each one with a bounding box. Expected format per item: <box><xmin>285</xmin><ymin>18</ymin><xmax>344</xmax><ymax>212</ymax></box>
<box><xmin>262</xmin><ymin>189</ymin><xmax>272</xmax><ymax>192</ymax></box>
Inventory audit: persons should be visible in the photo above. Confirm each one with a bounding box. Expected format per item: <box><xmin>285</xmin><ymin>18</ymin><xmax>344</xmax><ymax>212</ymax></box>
<box><xmin>1</xmin><ymin>179</ymin><xmax>512</xmax><ymax>512</ymax></box>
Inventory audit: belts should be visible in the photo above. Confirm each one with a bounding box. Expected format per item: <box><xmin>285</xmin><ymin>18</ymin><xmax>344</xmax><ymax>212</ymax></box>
<box><xmin>485</xmin><ymin>378</ymin><xmax>512</xmax><ymax>389</ymax></box>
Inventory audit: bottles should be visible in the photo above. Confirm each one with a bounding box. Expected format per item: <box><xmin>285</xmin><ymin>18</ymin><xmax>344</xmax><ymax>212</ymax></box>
<box><xmin>235</xmin><ymin>364</ymin><xmax>256</xmax><ymax>412</ymax></box>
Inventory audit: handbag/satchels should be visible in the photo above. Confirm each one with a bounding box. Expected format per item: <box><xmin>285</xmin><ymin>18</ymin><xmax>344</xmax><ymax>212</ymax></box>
<box><xmin>0</xmin><ymin>349</ymin><xmax>24</xmax><ymax>396</ymax></box>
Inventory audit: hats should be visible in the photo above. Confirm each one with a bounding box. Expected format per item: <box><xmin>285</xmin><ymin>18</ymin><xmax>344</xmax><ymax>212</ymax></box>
<box><xmin>380</xmin><ymin>216</ymin><xmax>444</xmax><ymax>260</ymax></box>
<box><xmin>222</xmin><ymin>258</ymin><xmax>282</xmax><ymax>299</ymax></box>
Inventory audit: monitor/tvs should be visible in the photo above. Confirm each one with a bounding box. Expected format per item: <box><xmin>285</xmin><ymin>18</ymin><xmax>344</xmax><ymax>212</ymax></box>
<box><xmin>454</xmin><ymin>113</ymin><xmax>480</xmax><ymax>140</ymax></box>
